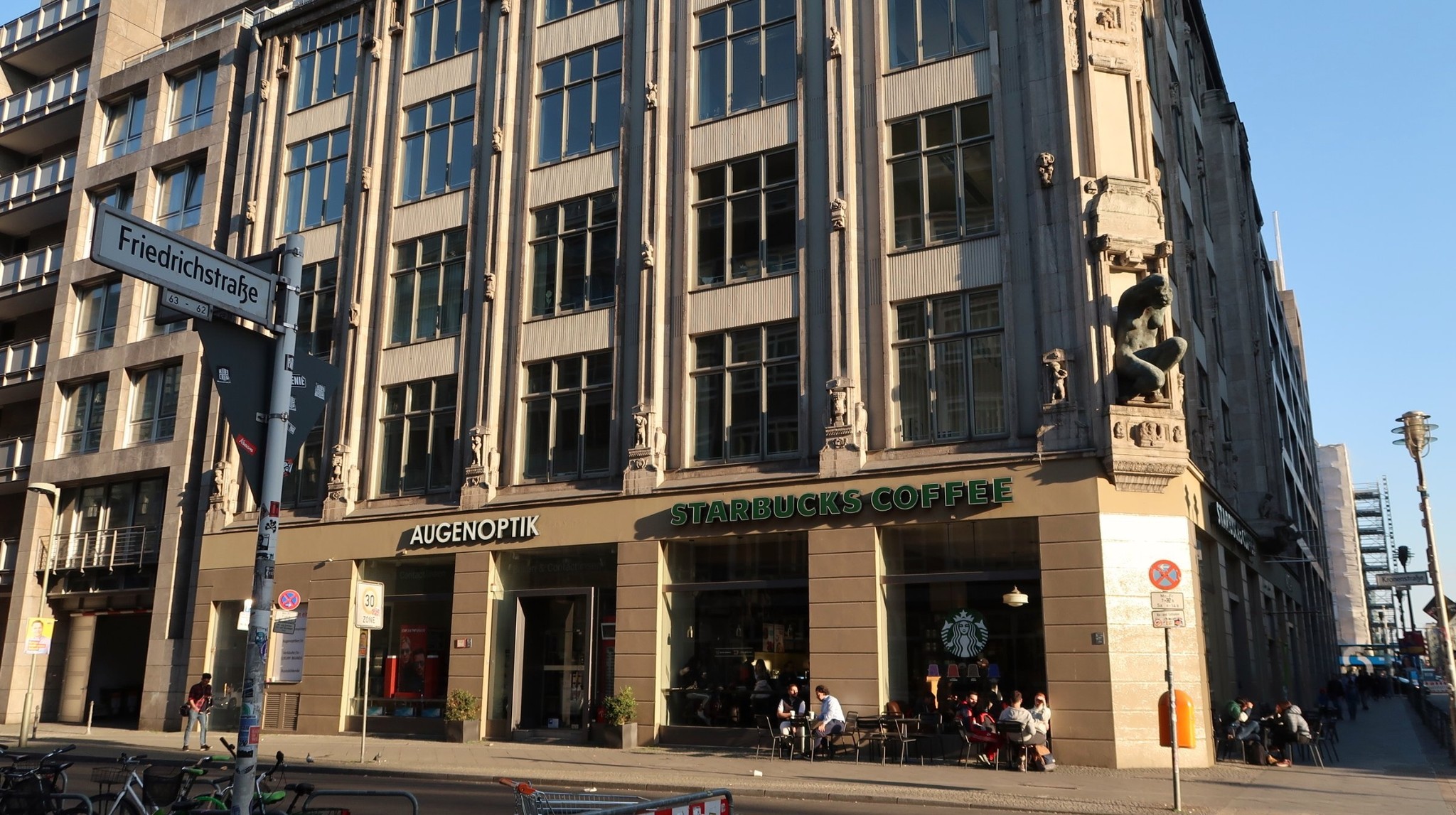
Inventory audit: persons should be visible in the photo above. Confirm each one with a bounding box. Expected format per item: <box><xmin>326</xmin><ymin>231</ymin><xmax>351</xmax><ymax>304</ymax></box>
<box><xmin>1221</xmin><ymin>694</ymin><xmax>1312</xmax><ymax>767</ymax></box>
<box><xmin>916</xmin><ymin>679</ymin><xmax>1010</xmax><ymax>733</ymax></box>
<box><xmin>492</xmin><ymin>127</ymin><xmax>501</xmax><ymax>144</ymax></box>
<box><xmin>182</xmin><ymin>673</ymin><xmax>213</xmax><ymax>751</ymax></box>
<box><xmin>226</xmin><ymin>684</ymin><xmax>234</xmax><ymax>693</ymax></box>
<box><xmin>1401</xmin><ymin>656</ymin><xmax>1419</xmax><ymax>680</ymax></box>
<box><xmin>635</xmin><ymin>415</ymin><xmax>648</xmax><ymax>448</ymax></box>
<box><xmin>1319</xmin><ymin>666</ymin><xmax>1399</xmax><ymax>723</ymax></box>
<box><xmin>215</xmin><ymin>470</ymin><xmax>223</xmax><ymax>495</ymax></box>
<box><xmin>644</xmin><ymin>240</ymin><xmax>653</xmax><ymax>257</ymax></box>
<box><xmin>680</xmin><ymin>653</ymin><xmax>798</xmax><ymax>720</ymax></box>
<box><xmin>1113</xmin><ymin>273</ymin><xmax>1187</xmax><ymax>403</ymax></box>
<box><xmin>25</xmin><ymin>621</ymin><xmax>51</xmax><ymax>654</ymax></box>
<box><xmin>803</xmin><ymin>685</ymin><xmax>845</xmax><ymax>761</ymax></box>
<box><xmin>998</xmin><ymin>690</ymin><xmax>1051</xmax><ymax>771</ymax></box>
<box><xmin>956</xmin><ymin>691</ymin><xmax>1006</xmax><ymax>766</ymax></box>
<box><xmin>776</xmin><ymin>683</ymin><xmax>805</xmax><ymax>750</ymax></box>
<box><xmin>1051</xmin><ymin>361</ymin><xmax>1068</xmax><ymax>400</ymax></box>
<box><xmin>398</xmin><ymin>637</ymin><xmax>426</xmax><ymax>692</ymax></box>
<box><xmin>472</xmin><ymin>437</ymin><xmax>482</xmax><ymax>467</ymax></box>
<box><xmin>1259</xmin><ymin>493</ymin><xmax>1274</xmax><ymax>519</ymax></box>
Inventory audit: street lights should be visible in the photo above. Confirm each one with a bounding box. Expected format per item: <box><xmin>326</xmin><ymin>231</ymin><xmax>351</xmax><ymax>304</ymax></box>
<box><xmin>1391</xmin><ymin>410</ymin><xmax>1456</xmax><ymax>691</ymax></box>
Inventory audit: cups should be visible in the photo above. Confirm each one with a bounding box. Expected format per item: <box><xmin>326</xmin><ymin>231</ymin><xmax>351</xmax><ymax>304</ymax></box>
<box><xmin>754</xmin><ymin>770</ymin><xmax>763</xmax><ymax>777</ymax></box>
<box><xmin>703</xmin><ymin>672</ymin><xmax>707</xmax><ymax>678</ymax></box>
<box><xmin>809</xmin><ymin>712</ymin><xmax>814</xmax><ymax>720</ymax></box>
<box><xmin>790</xmin><ymin>710</ymin><xmax>795</xmax><ymax>719</ymax></box>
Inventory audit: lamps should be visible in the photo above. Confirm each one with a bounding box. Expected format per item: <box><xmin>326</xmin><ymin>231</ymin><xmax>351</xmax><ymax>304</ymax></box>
<box><xmin>1003</xmin><ymin>585</ymin><xmax>1029</xmax><ymax>607</ymax></box>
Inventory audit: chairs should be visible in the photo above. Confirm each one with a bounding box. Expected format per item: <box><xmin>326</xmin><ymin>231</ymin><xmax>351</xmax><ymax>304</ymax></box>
<box><xmin>1211</xmin><ymin>708</ymin><xmax>1341</xmax><ymax>769</ymax></box>
<box><xmin>754</xmin><ymin>715</ymin><xmax>946</xmax><ymax>767</ymax></box>
<box><xmin>954</xmin><ymin>717</ymin><xmax>1050</xmax><ymax>773</ymax></box>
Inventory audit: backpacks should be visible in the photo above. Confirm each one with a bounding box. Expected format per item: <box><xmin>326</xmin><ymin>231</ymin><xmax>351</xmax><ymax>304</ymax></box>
<box><xmin>1247</xmin><ymin>742</ymin><xmax>1266</xmax><ymax>765</ymax></box>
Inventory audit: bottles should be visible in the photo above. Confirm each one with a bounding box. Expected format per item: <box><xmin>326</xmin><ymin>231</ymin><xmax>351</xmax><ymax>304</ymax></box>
<box><xmin>694</xmin><ymin>681</ymin><xmax>697</xmax><ymax>689</ymax></box>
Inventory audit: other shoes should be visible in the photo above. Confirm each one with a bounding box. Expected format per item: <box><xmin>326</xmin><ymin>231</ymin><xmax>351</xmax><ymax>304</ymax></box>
<box><xmin>975</xmin><ymin>752</ymin><xmax>991</xmax><ymax>768</ymax></box>
<box><xmin>797</xmin><ymin>751</ymin><xmax>812</xmax><ymax>760</ymax></box>
<box><xmin>183</xmin><ymin>746</ymin><xmax>189</xmax><ymax>752</ymax></box>
<box><xmin>1276</xmin><ymin>759</ymin><xmax>1291</xmax><ymax>768</ymax></box>
<box><xmin>785</xmin><ymin>737</ymin><xmax>791</xmax><ymax>748</ymax></box>
<box><xmin>1268</xmin><ymin>754</ymin><xmax>1280</xmax><ymax>765</ymax></box>
<box><xmin>201</xmin><ymin>745</ymin><xmax>213</xmax><ymax>751</ymax></box>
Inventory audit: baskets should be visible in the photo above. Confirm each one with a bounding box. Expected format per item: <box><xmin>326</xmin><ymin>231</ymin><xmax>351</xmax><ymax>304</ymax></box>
<box><xmin>91</xmin><ymin>767</ymin><xmax>133</xmax><ymax>784</ymax></box>
<box><xmin>13</xmin><ymin>760</ymin><xmax>40</xmax><ymax>784</ymax></box>
<box><xmin>143</xmin><ymin>765</ymin><xmax>185</xmax><ymax>807</ymax></box>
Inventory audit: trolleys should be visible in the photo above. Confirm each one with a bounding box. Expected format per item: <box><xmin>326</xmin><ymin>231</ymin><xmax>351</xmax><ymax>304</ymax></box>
<box><xmin>499</xmin><ymin>778</ymin><xmax>651</xmax><ymax>815</ymax></box>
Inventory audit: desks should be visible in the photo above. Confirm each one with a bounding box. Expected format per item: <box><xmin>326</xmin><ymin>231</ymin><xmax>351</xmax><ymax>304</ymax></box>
<box><xmin>779</xmin><ymin>716</ymin><xmax>817</xmax><ymax>754</ymax></box>
<box><xmin>856</xmin><ymin>718</ymin><xmax>922</xmax><ymax>764</ymax></box>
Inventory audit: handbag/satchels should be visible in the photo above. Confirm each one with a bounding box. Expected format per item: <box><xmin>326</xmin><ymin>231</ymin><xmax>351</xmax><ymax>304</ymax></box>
<box><xmin>180</xmin><ymin>702</ymin><xmax>191</xmax><ymax>716</ymax></box>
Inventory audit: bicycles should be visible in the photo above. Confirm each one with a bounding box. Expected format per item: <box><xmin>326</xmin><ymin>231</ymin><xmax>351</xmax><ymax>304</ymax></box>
<box><xmin>0</xmin><ymin>736</ymin><xmax>315</xmax><ymax>815</ymax></box>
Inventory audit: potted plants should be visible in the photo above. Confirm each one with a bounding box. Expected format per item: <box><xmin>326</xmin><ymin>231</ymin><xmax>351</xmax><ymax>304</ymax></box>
<box><xmin>443</xmin><ymin>689</ymin><xmax>481</xmax><ymax>742</ymax></box>
<box><xmin>602</xmin><ymin>687</ymin><xmax>639</xmax><ymax>749</ymax></box>
